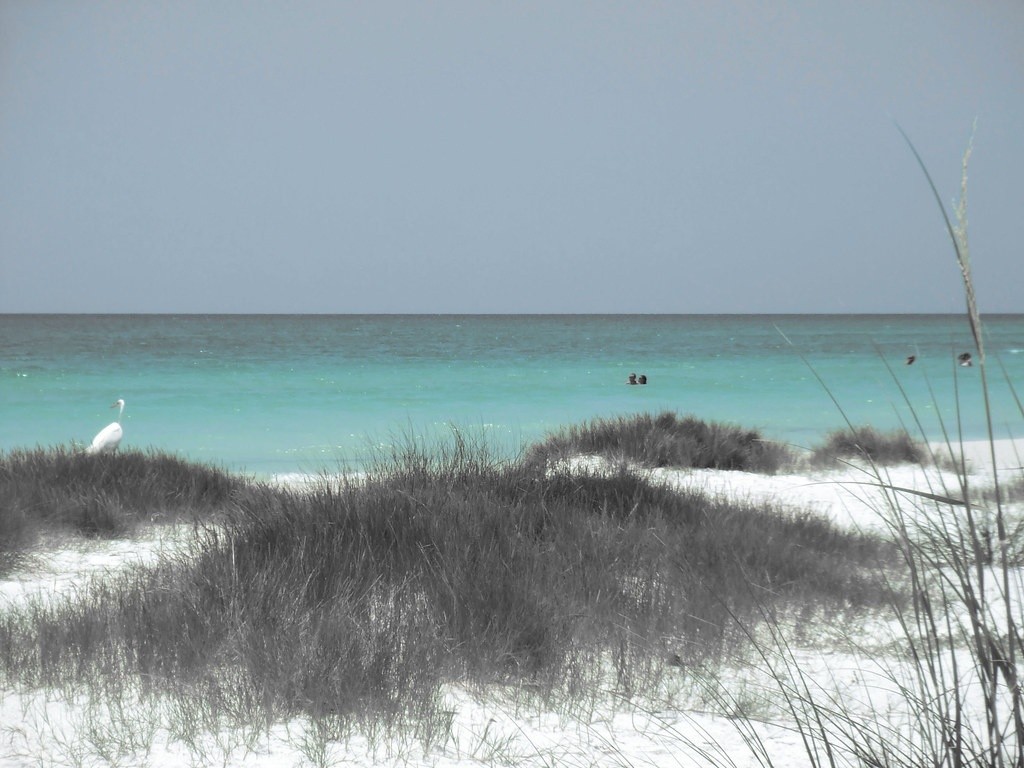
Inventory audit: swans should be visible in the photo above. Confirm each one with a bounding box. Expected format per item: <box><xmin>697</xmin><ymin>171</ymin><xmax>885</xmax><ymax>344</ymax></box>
<box><xmin>80</xmin><ymin>400</ymin><xmax>125</xmax><ymax>459</ymax></box>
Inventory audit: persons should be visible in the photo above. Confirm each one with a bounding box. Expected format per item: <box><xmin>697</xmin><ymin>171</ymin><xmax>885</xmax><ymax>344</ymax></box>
<box><xmin>626</xmin><ymin>373</ymin><xmax>636</xmax><ymax>384</ymax></box>
<box><xmin>905</xmin><ymin>356</ymin><xmax>915</xmax><ymax>364</ymax></box>
<box><xmin>958</xmin><ymin>352</ymin><xmax>972</xmax><ymax>367</ymax></box>
<box><xmin>638</xmin><ymin>375</ymin><xmax>647</xmax><ymax>384</ymax></box>
